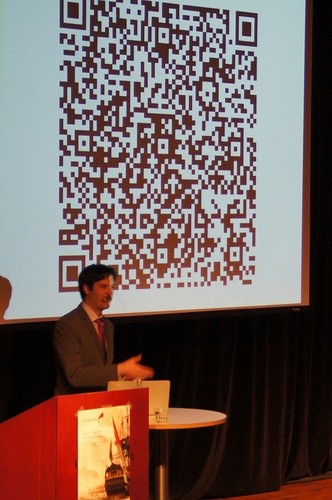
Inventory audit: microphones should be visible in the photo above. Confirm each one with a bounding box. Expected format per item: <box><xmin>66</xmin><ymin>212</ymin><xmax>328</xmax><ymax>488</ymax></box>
<box><xmin>97</xmin><ymin>297</ymin><xmax>106</xmax><ymax>301</ymax></box>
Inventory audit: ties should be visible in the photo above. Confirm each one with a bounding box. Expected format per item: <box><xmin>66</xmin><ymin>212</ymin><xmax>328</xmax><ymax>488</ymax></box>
<box><xmin>94</xmin><ymin>318</ymin><xmax>107</xmax><ymax>360</ymax></box>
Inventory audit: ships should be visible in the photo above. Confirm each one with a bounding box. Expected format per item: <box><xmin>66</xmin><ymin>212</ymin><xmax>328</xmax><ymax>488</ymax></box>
<box><xmin>99</xmin><ymin>407</ymin><xmax>131</xmax><ymax>496</ymax></box>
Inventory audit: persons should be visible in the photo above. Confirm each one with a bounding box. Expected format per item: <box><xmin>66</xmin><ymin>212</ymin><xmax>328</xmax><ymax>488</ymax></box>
<box><xmin>53</xmin><ymin>263</ymin><xmax>154</xmax><ymax>395</ymax></box>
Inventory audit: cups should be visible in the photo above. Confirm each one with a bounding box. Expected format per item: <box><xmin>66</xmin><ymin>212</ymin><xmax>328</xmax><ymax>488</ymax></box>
<box><xmin>154</xmin><ymin>407</ymin><xmax>169</xmax><ymax>423</ymax></box>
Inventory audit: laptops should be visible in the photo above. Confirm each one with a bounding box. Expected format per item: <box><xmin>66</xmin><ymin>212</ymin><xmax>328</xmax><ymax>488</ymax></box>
<box><xmin>107</xmin><ymin>380</ymin><xmax>170</xmax><ymax>416</ymax></box>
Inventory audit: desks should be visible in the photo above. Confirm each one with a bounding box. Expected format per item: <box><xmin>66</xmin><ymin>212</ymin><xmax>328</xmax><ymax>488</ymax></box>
<box><xmin>149</xmin><ymin>407</ymin><xmax>227</xmax><ymax>500</ymax></box>
